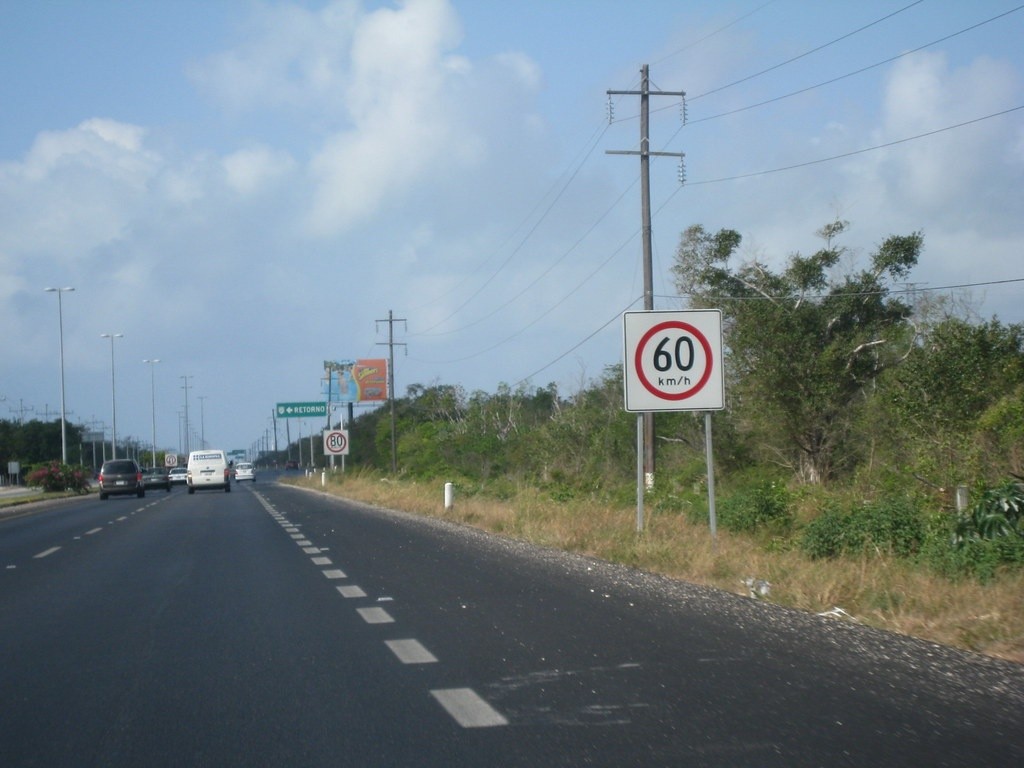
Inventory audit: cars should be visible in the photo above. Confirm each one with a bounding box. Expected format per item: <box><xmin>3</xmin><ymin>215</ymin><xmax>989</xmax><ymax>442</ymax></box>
<box><xmin>286</xmin><ymin>460</ymin><xmax>298</xmax><ymax>471</ymax></box>
<box><xmin>140</xmin><ymin>467</ymin><xmax>188</xmax><ymax>492</ymax></box>
<box><xmin>235</xmin><ymin>462</ymin><xmax>256</xmax><ymax>482</ymax></box>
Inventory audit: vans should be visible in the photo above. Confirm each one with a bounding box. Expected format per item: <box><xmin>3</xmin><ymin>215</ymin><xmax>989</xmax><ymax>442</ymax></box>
<box><xmin>183</xmin><ymin>449</ymin><xmax>233</xmax><ymax>495</ymax></box>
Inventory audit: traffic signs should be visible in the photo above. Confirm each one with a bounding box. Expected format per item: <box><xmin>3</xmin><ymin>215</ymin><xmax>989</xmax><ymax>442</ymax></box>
<box><xmin>277</xmin><ymin>401</ymin><xmax>327</xmax><ymax>418</ymax></box>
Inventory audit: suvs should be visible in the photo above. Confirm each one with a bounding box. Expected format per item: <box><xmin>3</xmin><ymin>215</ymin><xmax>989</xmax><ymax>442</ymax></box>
<box><xmin>98</xmin><ymin>458</ymin><xmax>148</xmax><ymax>500</ymax></box>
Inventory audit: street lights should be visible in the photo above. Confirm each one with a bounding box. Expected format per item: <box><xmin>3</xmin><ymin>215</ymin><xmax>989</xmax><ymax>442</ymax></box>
<box><xmin>44</xmin><ymin>287</ymin><xmax>75</xmax><ymax>464</ymax></box>
<box><xmin>178</xmin><ymin>375</ymin><xmax>195</xmax><ymax>459</ymax></box>
<box><xmin>196</xmin><ymin>394</ymin><xmax>207</xmax><ymax>451</ymax></box>
<box><xmin>100</xmin><ymin>332</ymin><xmax>124</xmax><ymax>460</ymax></box>
<box><xmin>143</xmin><ymin>359</ymin><xmax>160</xmax><ymax>474</ymax></box>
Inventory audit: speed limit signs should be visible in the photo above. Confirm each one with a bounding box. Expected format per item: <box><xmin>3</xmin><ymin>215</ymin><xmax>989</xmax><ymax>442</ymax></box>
<box><xmin>323</xmin><ymin>430</ymin><xmax>350</xmax><ymax>456</ymax></box>
<box><xmin>164</xmin><ymin>454</ymin><xmax>179</xmax><ymax>468</ymax></box>
<box><xmin>624</xmin><ymin>310</ymin><xmax>725</xmax><ymax>412</ymax></box>
